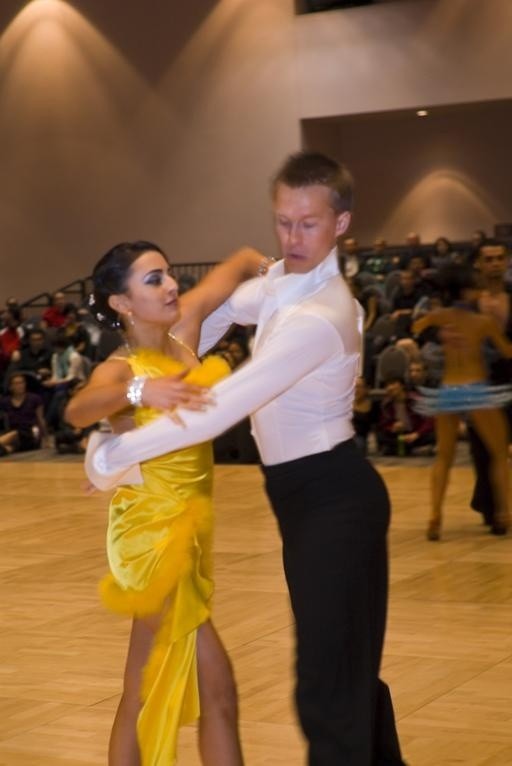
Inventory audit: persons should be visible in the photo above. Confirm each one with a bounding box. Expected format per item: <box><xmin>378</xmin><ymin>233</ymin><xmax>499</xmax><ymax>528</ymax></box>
<box><xmin>84</xmin><ymin>149</ymin><xmax>403</xmax><ymax>764</ymax></box>
<box><xmin>410</xmin><ymin>258</ymin><xmax>511</xmax><ymax>544</ymax></box>
<box><xmin>206</xmin><ymin>323</ymin><xmax>258</xmax><ymax>371</ymax></box>
<box><xmin>0</xmin><ymin>291</ymin><xmax>124</xmax><ymax>458</ymax></box>
<box><xmin>457</xmin><ymin>235</ymin><xmax>511</xmax><ymax>535</ymax></box>
<box><xmin>58</xmin><ymin>237</ymin><xmax>276</xmax><ymax>764</ymax></box>
<box><xmin>339</xmin><ymin>223</ymin><xmax>512</xmax><ymax>456</ymax></box>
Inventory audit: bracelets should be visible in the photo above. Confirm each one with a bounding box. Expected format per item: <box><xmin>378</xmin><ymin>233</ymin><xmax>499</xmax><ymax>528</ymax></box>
<box><xmin>126</xmin><ymin>371</ymin><xmax>147</xmax><ymax>408</ymax></box>
<box><xmin>257</xmin><ymin>256</ymin><xmax>275</xmax><ymax>277</ymax></box>
